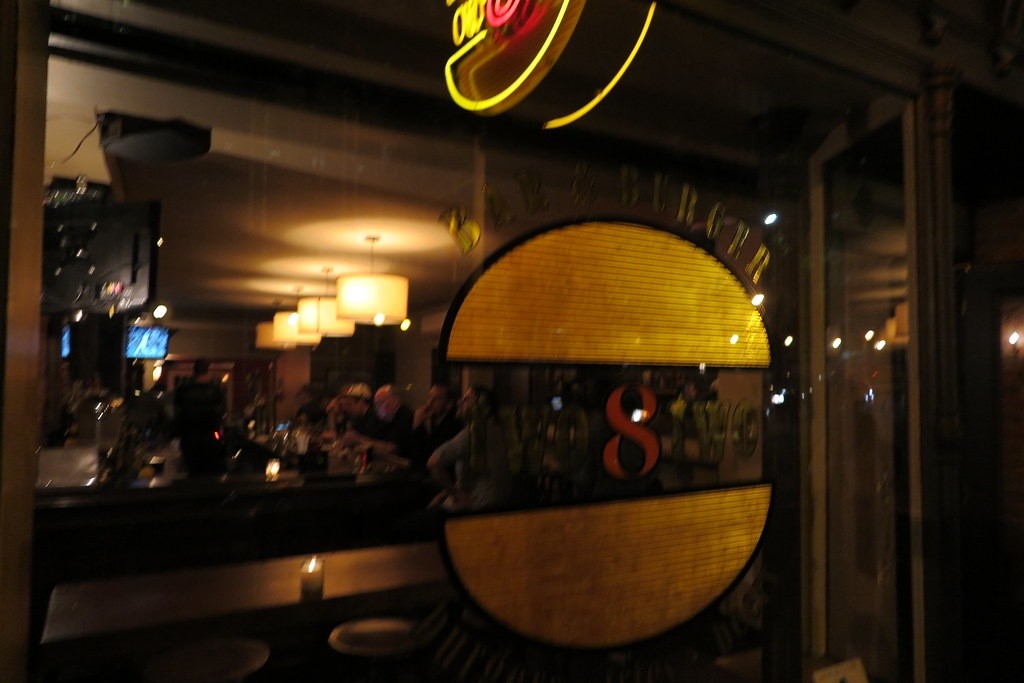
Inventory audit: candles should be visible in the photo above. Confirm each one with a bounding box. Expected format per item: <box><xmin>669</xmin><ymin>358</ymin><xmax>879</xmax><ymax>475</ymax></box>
<box><xmin>300</xmin><ymin>559</ymin><xmax>324</xmax><ymax>591</ymax></box>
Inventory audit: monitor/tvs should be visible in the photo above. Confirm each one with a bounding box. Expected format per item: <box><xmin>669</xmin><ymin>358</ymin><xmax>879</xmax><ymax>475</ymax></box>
<box><xmin>123</xmin><ymin>324</ymin><xmax>171</xmax><ymax>360</ymax></box>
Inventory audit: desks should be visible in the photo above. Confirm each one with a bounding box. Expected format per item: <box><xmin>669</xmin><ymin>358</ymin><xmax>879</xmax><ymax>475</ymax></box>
<box><xmin>38</xmin><ymin>543</ymin><xmax>452</xmax><ymax>683</ymax></box>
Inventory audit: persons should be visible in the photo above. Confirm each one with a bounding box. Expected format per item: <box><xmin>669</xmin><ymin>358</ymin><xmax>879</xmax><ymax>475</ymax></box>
<box><xmin>44</xmin><ymin>357</ymin><xmax>718</xmax><ymax>514</ymax></box>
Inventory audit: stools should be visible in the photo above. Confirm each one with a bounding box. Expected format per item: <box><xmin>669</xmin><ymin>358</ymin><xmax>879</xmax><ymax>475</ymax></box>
<box><xmin>149</xmin><ymin>639</ymin><xmax>272</xmax><ymax>683</ymax></box>
<box><xmin>326</xmin><ymin>617</ymin><xmax>424</xmax><ymax>683</ymax></box>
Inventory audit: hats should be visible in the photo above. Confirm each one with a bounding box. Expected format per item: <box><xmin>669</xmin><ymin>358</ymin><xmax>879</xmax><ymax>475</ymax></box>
<box><xmin>340</xmin><ymin>382</ymin><xmax>372</xmax><ymax>400</ymax></box>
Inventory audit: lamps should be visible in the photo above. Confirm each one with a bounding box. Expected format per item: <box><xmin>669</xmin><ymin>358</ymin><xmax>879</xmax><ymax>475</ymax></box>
<box><xmin>256</xmin><ymin>321</ymin><xmax>294</xmax><ymax>350</ymax></box>
<box><xmin>335</xmin><ymin>237</ymin><xmax>408</xmax><ymax>327</ymax></box>
<box><xmin>273</xmin><ymin>310</ymin><xmax>321</xmax><ymax>346</ymax></box>
<box><xmin>296</xmin><ymin>268</ymin><xmax>356</xmax><ymax>338</ymax></box>
